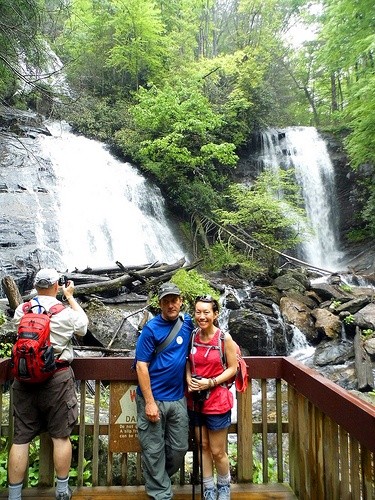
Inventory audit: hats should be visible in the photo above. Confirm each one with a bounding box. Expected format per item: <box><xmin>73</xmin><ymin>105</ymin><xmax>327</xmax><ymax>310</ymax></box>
<box><xmin>35</xmin><ymin>268</ymin><xmax>62</xmax><ymax>289</ymax></box>
<box><xmin>158</xmin><ymin>283</ymin><xmax>180</xmax><ymax>301</ymax></box>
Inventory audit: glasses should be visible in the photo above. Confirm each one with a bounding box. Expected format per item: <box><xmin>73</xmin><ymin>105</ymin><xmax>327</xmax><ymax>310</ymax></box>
<box><xmin>196</xmin><ymin>295</ymin><xmax>212</xmax><ymax>301</ymax></box>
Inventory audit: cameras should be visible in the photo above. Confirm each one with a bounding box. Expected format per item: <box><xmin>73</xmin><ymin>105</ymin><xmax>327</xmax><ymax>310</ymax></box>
<box><xmin>57</xmin><ymin>276</ymin><xmax>68</xmax><ymax>286</ymax></box>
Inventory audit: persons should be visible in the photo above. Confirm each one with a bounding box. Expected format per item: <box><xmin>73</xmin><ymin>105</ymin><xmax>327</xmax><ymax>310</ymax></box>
<box><xmin>132</xmin><ymin>282</ymin><xmax>194</xmax><ymax>500</ymax></box>
<box><xmin>184</xmin><ymin>296</ymin><xmax>237</xmax><ymax>500</ymax></box>
<box><xmin>8</xmin><ymin>268</ymin><xmax>89</xmax><ymax>500</ymax></box>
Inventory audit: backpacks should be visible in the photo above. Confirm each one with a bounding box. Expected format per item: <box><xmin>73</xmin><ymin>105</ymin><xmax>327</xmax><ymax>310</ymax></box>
<box><xmin>192</xmin><ymin>327</ymin><xmax>248</xmax><ymax>393</ymax></box>
<box><xmin>11</xmin><ymin>301</ymin><xmax>69</xmax><ymax>382</ymax></box>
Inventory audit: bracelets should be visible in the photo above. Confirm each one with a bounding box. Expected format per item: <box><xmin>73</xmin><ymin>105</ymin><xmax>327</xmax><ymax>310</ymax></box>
<box><xmin>209</xmin><ymin>378</ymin><xmax>211</xmax><ymax>388</ymax></box>
<box><xmin>212</xmin><ymin>377</ymin><xmax>217</xmax><ymax>386</ymax></box>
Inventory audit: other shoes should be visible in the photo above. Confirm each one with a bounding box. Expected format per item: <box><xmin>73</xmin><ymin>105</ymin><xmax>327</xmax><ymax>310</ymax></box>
<box><xmin>55</xmin><ymin>485</ymin><xmax>72</xmax><ymax>500</ymax></box>
<box><xmin>203</xmin><ymin>479</ymin><xmax>231</xmax><ymax>500</ymax></box>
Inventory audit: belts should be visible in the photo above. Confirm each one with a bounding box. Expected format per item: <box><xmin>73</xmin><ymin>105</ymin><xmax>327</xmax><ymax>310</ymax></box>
<box><xmin>55</xmin><ymin>361</ymin><xmax>70</xmax><ymax>370</ymax></box>
